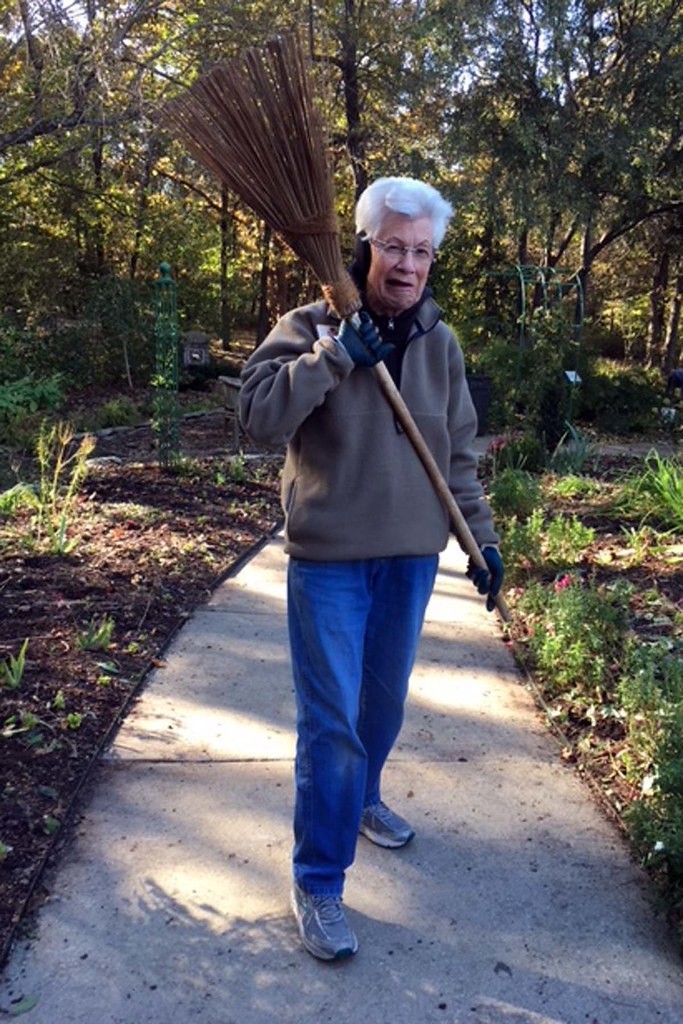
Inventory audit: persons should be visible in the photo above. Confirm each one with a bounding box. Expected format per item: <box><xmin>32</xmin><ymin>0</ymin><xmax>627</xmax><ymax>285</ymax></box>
<box><xmin>238</xmin><ymin>178</ymin><xmax>503</xmax><ymax>960</ymax></box>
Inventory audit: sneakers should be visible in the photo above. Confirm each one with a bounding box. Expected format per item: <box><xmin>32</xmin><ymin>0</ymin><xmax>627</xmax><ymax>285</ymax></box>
<box><xmin>290</xmin><ymin>879</ymin><xmax>358</xmax><ymax>960</ymax></box>
<box><xmin>358</xmin><ymin>801</ymin><xmax>416</xmax><ymax>849</ymax></box>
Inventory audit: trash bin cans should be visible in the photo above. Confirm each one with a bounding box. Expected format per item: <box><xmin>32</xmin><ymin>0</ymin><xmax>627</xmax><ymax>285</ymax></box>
<box><xmin>466</xmin><ymin>373</ymin><xmax>494</xmax><ymax>436</ymax></box>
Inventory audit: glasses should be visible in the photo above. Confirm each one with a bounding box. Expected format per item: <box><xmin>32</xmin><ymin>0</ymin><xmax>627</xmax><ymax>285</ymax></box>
<box><xmin>366</xmin><ymin>234</ymin><xmax>436</xmax><ymax>262</ymax></box>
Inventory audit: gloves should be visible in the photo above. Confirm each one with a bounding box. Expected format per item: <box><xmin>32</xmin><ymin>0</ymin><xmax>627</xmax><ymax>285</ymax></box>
<box><xmin>335</xmin><ymin>311</ymin><xmax>395</xmax><ymax>369</ymax></box>
<box><xmin>464</xmin><ymin>547</ymin><xmax>504</xmax><ymax>612</ymax></box>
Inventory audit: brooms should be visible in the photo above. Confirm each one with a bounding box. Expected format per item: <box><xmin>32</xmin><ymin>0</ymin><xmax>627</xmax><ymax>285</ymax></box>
<box><xmin>147</xmin><ymin>23</ymin><xmax>511</xmax><ymax>623</ymax></box>
